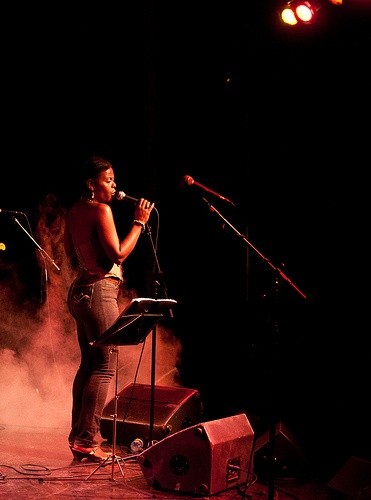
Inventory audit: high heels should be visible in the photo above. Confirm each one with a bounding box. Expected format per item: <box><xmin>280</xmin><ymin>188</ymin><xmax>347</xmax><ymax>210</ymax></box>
<box><xmin>69</xmin><ymin>443</ymin><xmax>117</xmax><ymax>462</ymax></box>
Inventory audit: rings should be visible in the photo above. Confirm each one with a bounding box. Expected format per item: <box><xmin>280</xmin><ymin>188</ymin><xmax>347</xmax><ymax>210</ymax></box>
<box><xmin>146</xmin><ymin>205</ymin><xmax>149</xmax><ymax>208</ymax></box>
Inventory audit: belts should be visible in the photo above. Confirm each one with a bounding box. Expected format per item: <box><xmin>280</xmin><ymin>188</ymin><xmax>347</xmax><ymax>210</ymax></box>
<box><xmin>106</xmin><ymin>277</ymin><xmax>121</xmax><ymax>285</ymax></box>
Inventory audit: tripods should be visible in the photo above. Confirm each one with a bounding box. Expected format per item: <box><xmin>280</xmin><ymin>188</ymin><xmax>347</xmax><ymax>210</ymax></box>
<box><xmin>85</xmin><ymin>298</ymin><xmax>177</xmax><ymax>481</ymax></box>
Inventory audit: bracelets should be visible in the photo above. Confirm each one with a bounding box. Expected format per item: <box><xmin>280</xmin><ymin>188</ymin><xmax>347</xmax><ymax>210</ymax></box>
<box><xmin>132</xmin><ymin>220</ymin><xmax>146</xmax><ymax>231</ymax></box>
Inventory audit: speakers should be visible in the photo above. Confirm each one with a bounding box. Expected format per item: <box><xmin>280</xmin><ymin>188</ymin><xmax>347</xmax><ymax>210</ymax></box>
<box><xmin>98</xmin><ymin>364</ymin><xmax>304</xmax><ymax>495</ymax></box>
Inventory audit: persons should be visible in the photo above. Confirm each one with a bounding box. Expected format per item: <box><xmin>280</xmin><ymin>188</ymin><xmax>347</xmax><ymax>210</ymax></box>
<box><xmin>67</xmin><ymin>158</ymin><xmax>155</xmax><ymax>463</ymax></box>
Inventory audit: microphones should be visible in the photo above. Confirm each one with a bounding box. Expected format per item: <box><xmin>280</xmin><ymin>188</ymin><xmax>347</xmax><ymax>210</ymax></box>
<box><xmin>0</xmin><ymin>208</ymin><xmax>23</xmax><ymax>215</ymax></box>
<box><xmin>180</xmin><ymin>175</ymin><xmax>235</xmax><ymax>208</ymax></box>
<box><xmin>115</xmin><ymin>190</ymin><xmax>152</xmax><ymax>207</ymax></box>
